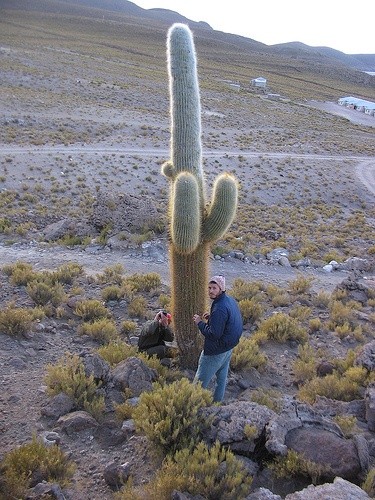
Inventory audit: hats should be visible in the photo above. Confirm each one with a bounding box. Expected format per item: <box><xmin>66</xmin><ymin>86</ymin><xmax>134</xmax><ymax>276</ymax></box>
<box><xmin>208</xmin><ymin>275</ymin><xmax>226</xmax><ymax>292</ymax></box>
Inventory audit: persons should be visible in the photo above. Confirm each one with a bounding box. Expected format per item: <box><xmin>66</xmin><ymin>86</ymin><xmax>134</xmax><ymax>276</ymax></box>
<box><xmin>137</xmin><ymin>311</ymin><xmax>175</xmax><ymax>359</ymax></box>
<box><xmin>193</xmin><ymin>274</ymin><xmax>243</xmax><ymax>406</ymax></box>
